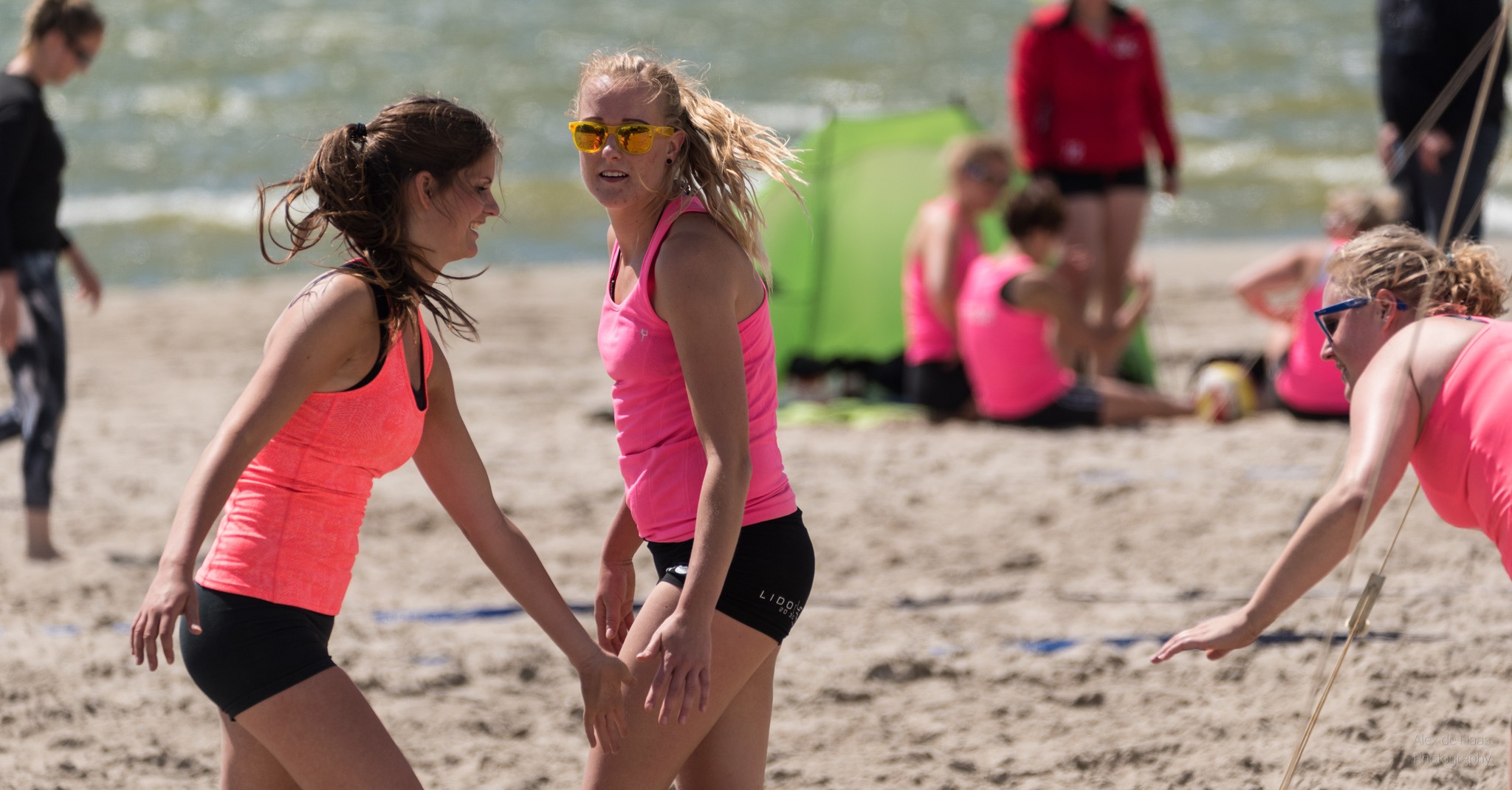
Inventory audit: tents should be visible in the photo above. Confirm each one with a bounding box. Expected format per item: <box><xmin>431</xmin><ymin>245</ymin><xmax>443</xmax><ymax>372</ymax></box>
<box><xmin>736</xmin><ymin>93</ymin><xmax>1158</xmax><ymax>410</ymax></box>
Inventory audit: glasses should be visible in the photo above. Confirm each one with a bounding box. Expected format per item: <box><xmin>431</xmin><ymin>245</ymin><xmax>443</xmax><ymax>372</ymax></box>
<box><xmin>568</xmin><ymin>122</ymin><xmax>675</xmax><ymax>155</ymax></box>
<box><xmin>1312</xmin><ymin>296</ymin><xmax>1409</xmax><ymax>346</ymax></box>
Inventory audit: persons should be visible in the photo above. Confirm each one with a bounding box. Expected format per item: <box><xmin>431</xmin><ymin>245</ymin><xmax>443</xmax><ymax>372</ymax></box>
<box><xmin>574</xmin><ymin>53</ymin><xmax>819</xmax><ymax>790</ymax></box>
<box><xmin>128</xmin><ymin>88</ymin><xmax>638</xmax><ymax>790</ymax></box>
<box><xmin>900</xmin><ymin>0</ymin><xmax>1512</xmax><ymax>666</ymax></box>
<box><xmin>0</xmin><ymin>0</ymin><xmax>104</xmax><ymax>565</ymax></box>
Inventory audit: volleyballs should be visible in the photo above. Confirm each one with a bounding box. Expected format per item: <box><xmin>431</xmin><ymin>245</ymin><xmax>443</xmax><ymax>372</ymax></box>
<box><xmin>1193</xmin><ymin>359</ymin><xmax>1259</xmax><ymax>427</ymax></box>
